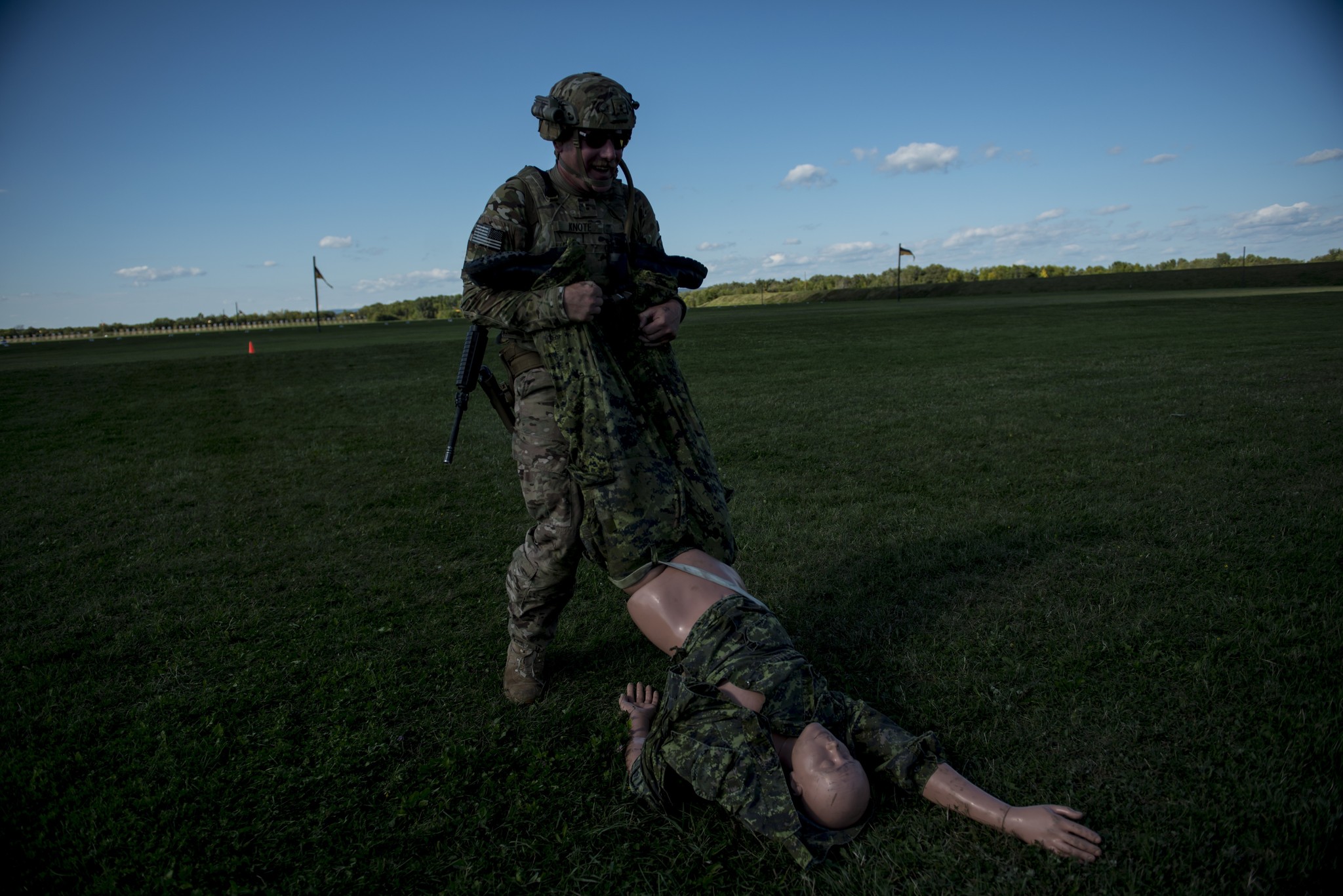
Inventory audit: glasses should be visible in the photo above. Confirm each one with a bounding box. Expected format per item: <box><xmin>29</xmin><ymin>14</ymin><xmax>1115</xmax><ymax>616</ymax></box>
<box><xmin>577</xmin><ymin>125</ymin><xmax>631</xmax><ymax>151</ymax></box>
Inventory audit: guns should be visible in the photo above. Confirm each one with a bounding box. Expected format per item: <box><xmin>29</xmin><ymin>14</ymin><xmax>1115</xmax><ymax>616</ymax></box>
<box><xmin>444</xmin><ymin>321</ymin><xmax>516</xmax><ymax>465</ymax></box>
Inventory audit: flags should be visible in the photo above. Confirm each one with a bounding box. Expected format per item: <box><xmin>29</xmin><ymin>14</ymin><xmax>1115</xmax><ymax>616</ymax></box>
<box><xmin>315</xmin><ymin>266</ymin><xmax>334</xmax><ymax>289</ymax></box>
<box><xmin>900</xmin><ymin>247</ymin><xmax>915</xmax><ymax>261</ymax></box>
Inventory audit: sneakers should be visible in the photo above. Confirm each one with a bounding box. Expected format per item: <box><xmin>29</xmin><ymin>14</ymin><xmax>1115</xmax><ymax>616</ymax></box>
<box><xmin>502</xmin><ymin>638</ymin><xmax>544</xmax><ymax>703</ymax></box>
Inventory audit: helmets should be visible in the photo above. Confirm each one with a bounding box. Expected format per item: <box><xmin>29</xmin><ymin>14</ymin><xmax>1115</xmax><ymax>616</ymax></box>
<box><xmin>530</xmin><ymin>72</ymin><xmax>638</xmax><ymax>139</ymax></box>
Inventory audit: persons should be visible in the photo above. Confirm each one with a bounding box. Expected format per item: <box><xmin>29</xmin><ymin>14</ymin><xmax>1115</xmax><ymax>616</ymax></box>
<box><xmin>464</xmin><ymin>252</ymin><xmax>1106</xmax><ymax>870</ymax></box>
<box><xmin>461</xmin><ymin>70</ymin><xmax>690</xmax><ymax>709</ymax></box>
